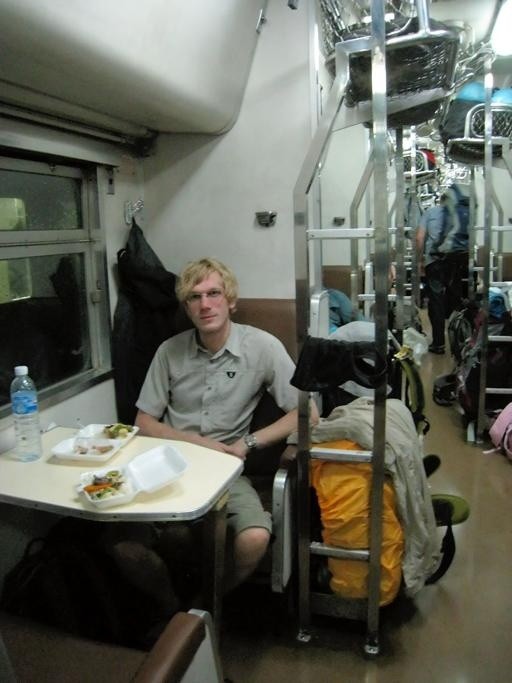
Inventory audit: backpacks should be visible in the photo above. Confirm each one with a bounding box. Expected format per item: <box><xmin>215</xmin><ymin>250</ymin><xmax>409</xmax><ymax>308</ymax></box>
<box><xmin>438</xmin><ymin>80</ymin><xmax>512</xmax><ymax>159</ymax></box>
<box><xmin>387</xmin><ymin>345</ymin><xmax>431</xmax><ymax>436</ymax></box>
<box><xmin>426</xmin><ymin>183</ymin><xmax>470</xmax><ymax>260</ymax></box>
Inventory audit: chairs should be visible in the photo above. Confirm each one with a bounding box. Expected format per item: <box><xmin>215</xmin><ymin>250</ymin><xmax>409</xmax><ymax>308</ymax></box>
<box><xmin>228</xmin><ymin>292</ymin><xmax>299</xmax><ymax>625</ymax></box>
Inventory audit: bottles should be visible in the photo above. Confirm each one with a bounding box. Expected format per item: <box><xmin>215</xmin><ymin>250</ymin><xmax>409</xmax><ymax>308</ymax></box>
<box><xmin>10</xmin><ymin>365</ymin><xmax>43</xmax><ymax>461</ymax></box>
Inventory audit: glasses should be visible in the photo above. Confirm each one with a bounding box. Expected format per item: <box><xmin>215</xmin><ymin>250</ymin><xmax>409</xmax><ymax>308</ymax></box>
<box><xmin>188</xmin><ymin>287</ymin><xmax>222</xmax><ymax>300</ymax></box>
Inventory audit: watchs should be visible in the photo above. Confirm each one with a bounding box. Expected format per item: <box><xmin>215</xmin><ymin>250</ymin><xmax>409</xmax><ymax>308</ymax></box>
<box><xmin>244</xmin><ymin>434</ymin><xmax>257</xmax><ymax>453</ymax></box>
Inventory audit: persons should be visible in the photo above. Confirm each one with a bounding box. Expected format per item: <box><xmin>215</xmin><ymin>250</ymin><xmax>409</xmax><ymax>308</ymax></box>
<box><xmin>417</xmin><ymin>194</ymin><xmax>468</xmax><ymax>355</ymax></box>
<box><xmin>102</xmin><ymin>259</ymin><xmax>320</xmax><ymax>643</ymax></box>
